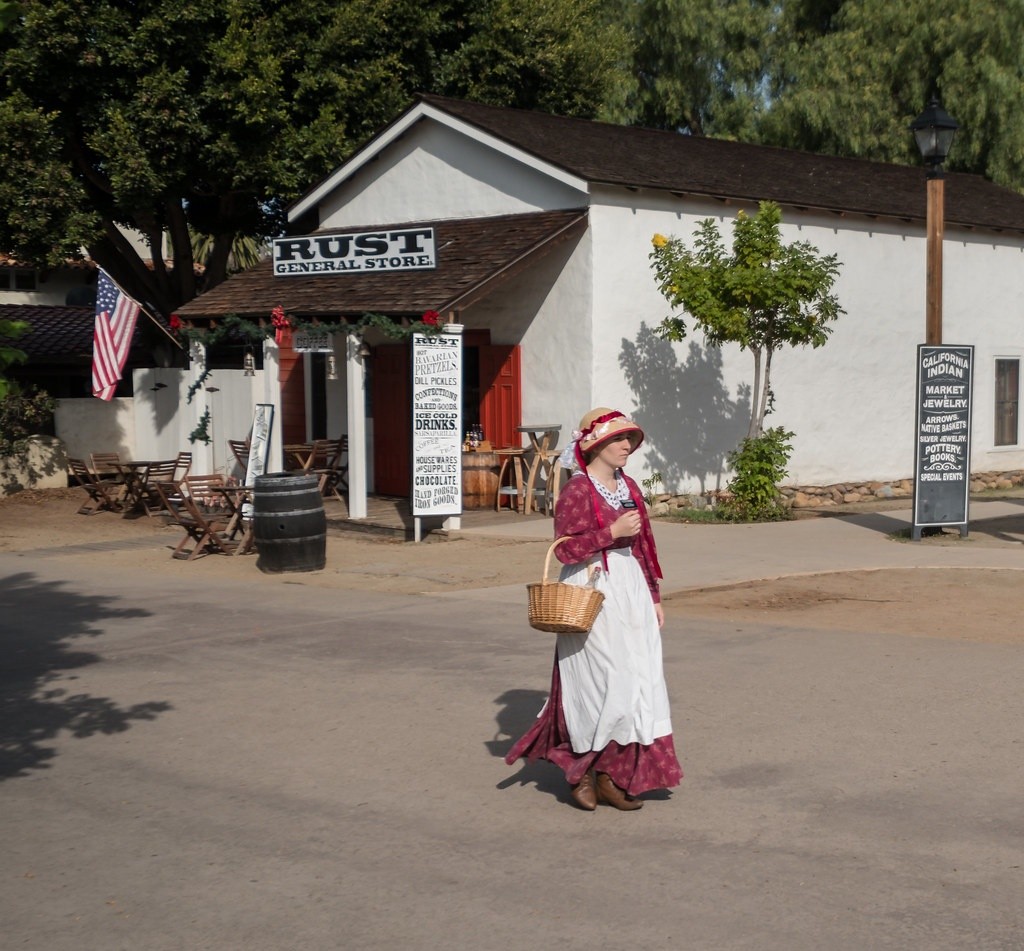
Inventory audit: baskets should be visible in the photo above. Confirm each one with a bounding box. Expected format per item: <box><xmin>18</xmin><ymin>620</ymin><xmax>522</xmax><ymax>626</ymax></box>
<box><xmin>525</xmin><ymin>537</ymin><xmax>605</xmax><ymax>633</ymax></box>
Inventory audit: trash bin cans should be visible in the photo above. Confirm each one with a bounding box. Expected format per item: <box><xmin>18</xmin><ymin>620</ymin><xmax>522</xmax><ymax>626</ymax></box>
<box><xmin>255</xmin><ymin>470</ymin><xmax>327</xmax><ymax>574</ymax></box>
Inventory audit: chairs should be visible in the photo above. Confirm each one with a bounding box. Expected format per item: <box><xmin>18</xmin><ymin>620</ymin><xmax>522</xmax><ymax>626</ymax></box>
<box><xmin>67</xmin><ymin>432</ymin><xmax>348</xmax><ymax>561</ymax></box>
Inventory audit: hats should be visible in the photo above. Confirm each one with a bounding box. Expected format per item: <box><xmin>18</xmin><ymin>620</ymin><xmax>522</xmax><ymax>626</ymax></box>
<box><xmin>571</xmin><ymin>408</ymin><xmax>644</xmax><ymax>456</ymax></box>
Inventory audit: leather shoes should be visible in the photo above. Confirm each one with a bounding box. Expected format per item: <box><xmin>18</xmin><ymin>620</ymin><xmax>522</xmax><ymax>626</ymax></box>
<box><xmin>570</xmin><ymin>766</ymin><xmax>596</xmax><ymax>810</ymax></box>
<box><xmin>595</xmin><ymin>771</ymin><xmax>643</xmax><ymax>810</ymax></box>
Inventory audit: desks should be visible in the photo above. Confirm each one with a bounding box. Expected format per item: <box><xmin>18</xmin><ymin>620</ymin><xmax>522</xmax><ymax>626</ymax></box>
<box><xmin>517</xmin><ymin>423</ymin><xmax>561</xmax><ymax>515</ymax></box>
<box><xmin>209</xmin><ymin>485</ymin><xmax>259</xmax><ymax>555</ymax></box>
<box><xmin>105</xmin><ymin>461</ymin><xmax>160</xmax><ymax>518</ymax></box>
<box><xmin>460</xmin><ymin>451</ymin><xmax>502</xmax><ymax>510</ymax></box>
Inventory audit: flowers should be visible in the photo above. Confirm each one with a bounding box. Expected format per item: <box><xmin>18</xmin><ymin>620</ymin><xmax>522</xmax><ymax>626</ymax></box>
<box><xmin>168</xmin><ymin>314</ymin><xmax>184</xmax><ymax>332</ymax></box>
<box><xmin>425</xmin><ymin>309</ymin><xmax>441</xmax><ymax>330</ymax></box>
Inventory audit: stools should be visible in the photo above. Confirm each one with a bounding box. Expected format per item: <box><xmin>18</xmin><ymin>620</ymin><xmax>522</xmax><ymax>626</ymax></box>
<box><xmin>491</xmin><ymin>448</ymin><xmax>540</xmax><ymax>512</ymax></box>
<box><xmin>544</xmin><ymin>450</ymin><xmax>574</xmax><ymax>518</ymax></box>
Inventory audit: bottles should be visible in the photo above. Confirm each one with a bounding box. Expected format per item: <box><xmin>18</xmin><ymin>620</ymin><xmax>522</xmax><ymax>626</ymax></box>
<box><xmin>582</xmin><ymin>566</ymin><xmax>602</xmax><ymax>591</ymax></box>
<box><xmin>461</xmin><ymin>424</ymin><xmax>485</xmax><ymax>452</ymax></box>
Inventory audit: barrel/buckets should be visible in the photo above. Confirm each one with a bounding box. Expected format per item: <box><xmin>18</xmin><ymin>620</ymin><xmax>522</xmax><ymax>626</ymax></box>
<box><xmin>462</xmin><ymin>452</ymin><xmax>502</xmax><ymax>510</ymax></box>
<box><xmin>253</xmin><ymin>471</ymin><xmax>328</xmax><ymax>573</ymax></box>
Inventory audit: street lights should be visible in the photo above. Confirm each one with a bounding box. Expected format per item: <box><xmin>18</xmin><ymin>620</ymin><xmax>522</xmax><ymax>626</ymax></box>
<box><xmin>909</xmin><ymin>82</ymin><xmax>961</xmax><ymax>534</ymax></box>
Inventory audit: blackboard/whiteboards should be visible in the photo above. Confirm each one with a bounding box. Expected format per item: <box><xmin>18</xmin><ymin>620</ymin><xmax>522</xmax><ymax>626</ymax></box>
<box><xmin>913</xmin><ymin>342</ymin><xmax>975</xmax><ymax>540</ymax></box>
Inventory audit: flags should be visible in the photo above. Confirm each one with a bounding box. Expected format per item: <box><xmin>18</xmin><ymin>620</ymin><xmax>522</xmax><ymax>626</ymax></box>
<box><xmin>92</xmin><ymin>270</ymin><xmax>140</xmax><ymax>401</ymax></box>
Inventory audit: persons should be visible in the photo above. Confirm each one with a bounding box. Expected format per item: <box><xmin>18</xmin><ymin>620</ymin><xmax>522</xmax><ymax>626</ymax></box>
<box><xmin>552</xmin><ymin>408</ymin><xmax>667</xmax><ymax>811</ymax></box>
